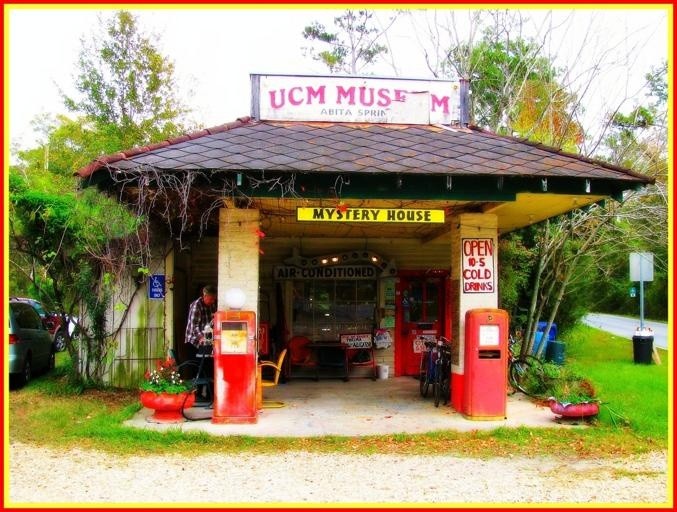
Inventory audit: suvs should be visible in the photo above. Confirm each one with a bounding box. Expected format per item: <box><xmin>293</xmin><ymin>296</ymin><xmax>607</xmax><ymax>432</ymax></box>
<box><xmin>8</xmin><ymin>296</ymin><xmax>67</xmax><ymax>353</ymax></box>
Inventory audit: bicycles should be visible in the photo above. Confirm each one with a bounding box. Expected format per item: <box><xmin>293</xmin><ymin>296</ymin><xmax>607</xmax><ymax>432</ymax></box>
<box><xmin>415</xmin><ymin>329</ymin><xmax>548</xmax><ymax>411</ymax></box>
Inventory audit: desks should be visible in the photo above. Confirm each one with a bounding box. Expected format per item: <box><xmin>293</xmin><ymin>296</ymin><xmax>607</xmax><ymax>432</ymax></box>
<box><xmin>196</xmin><ymin>352</ymin><xmax>213</xmax><ymax>409</ymax></box>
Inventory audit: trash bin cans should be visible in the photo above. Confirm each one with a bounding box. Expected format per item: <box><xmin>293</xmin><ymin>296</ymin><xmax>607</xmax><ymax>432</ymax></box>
<box><xmin>532</xmin><ymin>321</ymin><xmax>565</xmax><ymax>366</ymax></box>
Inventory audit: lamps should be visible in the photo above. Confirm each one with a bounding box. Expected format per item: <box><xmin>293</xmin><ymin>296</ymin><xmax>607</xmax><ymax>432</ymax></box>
<box><xmin>282</xmin><ymin>235</ymin><xmax>398</xmax><ymax>280</ymax></box>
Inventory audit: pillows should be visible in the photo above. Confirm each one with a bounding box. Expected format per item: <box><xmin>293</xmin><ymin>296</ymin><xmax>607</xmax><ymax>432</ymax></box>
<box><xmin>352</xmin><ymin>350</ymin><xmax>370</xmax><ymax>363</ymax></box>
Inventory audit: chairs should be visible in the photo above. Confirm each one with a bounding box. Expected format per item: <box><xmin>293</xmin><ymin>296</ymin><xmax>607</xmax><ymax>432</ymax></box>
<box><xmin>256</xmin><ymin>348</ymin><xmax>290</xmax><ymax>409</ymax></box>
<box><xmin>167</xmin><ymin>348</ymin><xmax>212</xmax><ymax>405</ymax></box>
<box><xmin>284</xmin><ymin>336</ymin><xmax>317</xmax><ymax>381</ymax></box>
<box><xmin>345</xmin><ymin>341</ymin><xmax>374</xmax><ymax>380</ymax></box>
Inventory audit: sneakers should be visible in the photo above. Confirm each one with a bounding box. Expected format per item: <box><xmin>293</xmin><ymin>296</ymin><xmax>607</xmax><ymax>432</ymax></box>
<box><xmin>196</xmin><ymin>395</ymin><xmax>206</xmax><ymax>402</ymax></box>
<box><xmin>206</xmin><ymin>395</ymin><xmax>211</xmax><ymax>402</ymax></box>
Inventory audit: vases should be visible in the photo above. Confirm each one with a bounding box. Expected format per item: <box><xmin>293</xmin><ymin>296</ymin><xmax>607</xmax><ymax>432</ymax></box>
<box><xmin>549</xmin><ymin>399</ymin><xmax>598</xmax><ymax>423</ymax></box>
<box><xmin>139</xmin><ymin>390</ymin><xmax>195</xmax><ymax>419</ymax></box>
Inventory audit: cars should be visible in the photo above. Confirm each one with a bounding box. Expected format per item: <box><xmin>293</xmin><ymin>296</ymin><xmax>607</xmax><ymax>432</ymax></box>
<box><xmin>8</xmin><ymin>300</ymin><xmax>56</xmax><ymax>389</ymax></box>
<box><xmin>54</xmin><ymin>313</ymin><xmax>79</xmax><ymax>340</ymax></box>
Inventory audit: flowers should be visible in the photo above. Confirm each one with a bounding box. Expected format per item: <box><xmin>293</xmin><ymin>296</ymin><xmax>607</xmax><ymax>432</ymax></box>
<box><xmin>138</xmin><ymin>354</ymin><xmax>198</xmax><ymax>394</ymax></box>
<box><xmin>549</xmin><ymin>376</ymin><xmax>599</xmax><ymax>405</ymax></box>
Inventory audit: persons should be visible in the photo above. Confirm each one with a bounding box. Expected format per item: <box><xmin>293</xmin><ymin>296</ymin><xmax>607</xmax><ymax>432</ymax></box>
<box><xmin>185</xmin><ymin>284</ymin><xmax>217</xmax><ymax>402</ymax></box>
